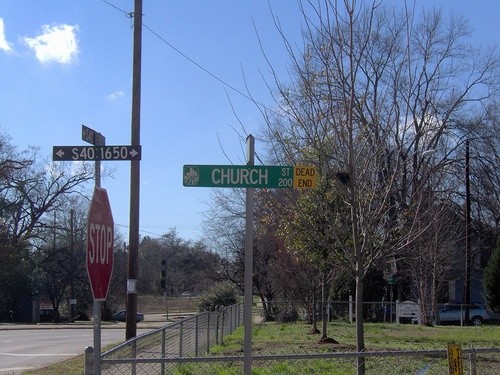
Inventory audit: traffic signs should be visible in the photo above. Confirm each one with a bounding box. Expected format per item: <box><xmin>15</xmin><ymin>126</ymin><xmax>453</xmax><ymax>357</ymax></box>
<box><xmin>182</xmin><ymin>165</ymin><xmax>317</xmax><ymax>189</ymax></box>
<box><xmin>51</xmin><ymin>145</ymin><xmax>141</xmax><ymax>161</ymax></box>
<box><xmin>82</xmin><ymin>124</ymin><xmax>106</xmax><ymax>148</ymax></box>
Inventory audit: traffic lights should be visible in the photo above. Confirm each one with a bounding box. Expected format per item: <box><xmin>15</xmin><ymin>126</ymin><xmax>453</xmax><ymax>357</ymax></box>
<box><xmin>160</xmin><ymin>260</ymin><xmax>169</xmax><ymax>289</ymax></box>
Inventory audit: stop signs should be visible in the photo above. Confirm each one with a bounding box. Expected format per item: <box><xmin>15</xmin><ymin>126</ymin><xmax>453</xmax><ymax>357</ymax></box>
<box><xmin>87</xmin><ymin>187</ymin><xmax>115</xmax><ymax>302</ymax></box>
